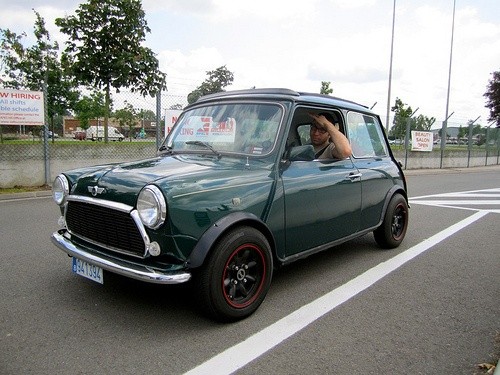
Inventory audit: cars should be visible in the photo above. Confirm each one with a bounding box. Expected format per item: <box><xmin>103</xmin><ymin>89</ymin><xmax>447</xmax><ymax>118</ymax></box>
<box><xmin>389</xmin><ymin>139</ymin><xmax>403</xmax><ymax>145</ymax></box>
<box><xmin>433</xmin><ymin>139</ymin><xmax>479</xmax><ymax>145</ymax></box>
<box><xmin>50</xmin><ymin>87</ymin><xmax>411</xmax><ymax>321</ymax></box>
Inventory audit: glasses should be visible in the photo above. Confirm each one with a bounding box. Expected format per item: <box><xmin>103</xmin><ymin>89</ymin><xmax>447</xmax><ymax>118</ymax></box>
<box><xmin>310</xmin><ymin>125</ymin><xmax>327</xmax><ymax>134</ymax></box>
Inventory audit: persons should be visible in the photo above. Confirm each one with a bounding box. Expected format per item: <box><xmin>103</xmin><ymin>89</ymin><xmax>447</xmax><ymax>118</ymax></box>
<box><xmin>306</xmin><ymin>111</ymin><xmax>352</xmax><ymax>160</ymax></box>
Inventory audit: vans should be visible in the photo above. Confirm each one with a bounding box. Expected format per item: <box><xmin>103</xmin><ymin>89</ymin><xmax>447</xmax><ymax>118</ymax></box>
<box><xmin>86</xmin><ymin>126</ymin><xmax>126</xmax><ymax>142</ymax></box>
